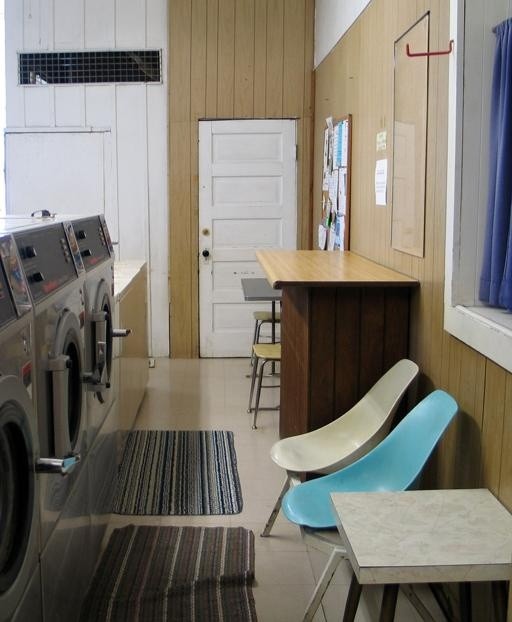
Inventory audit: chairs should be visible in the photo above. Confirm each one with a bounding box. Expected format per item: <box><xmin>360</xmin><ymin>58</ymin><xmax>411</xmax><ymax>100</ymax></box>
<box><xmin>261</xmin><ymin>358</ymin><xmax>461</xmax><ymax>622</ymax></box>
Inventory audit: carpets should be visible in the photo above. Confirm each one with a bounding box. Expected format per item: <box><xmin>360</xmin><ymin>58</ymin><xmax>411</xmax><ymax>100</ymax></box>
<box><xmin>83</xmin><ymin>427</ymin><xmax>260</xmax><ymax>621</ymax></box>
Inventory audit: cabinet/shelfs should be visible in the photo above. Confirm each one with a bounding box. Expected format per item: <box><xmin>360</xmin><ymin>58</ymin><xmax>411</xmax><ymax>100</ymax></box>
<box><xmin>257</xmin><ymin>252</ymin><xmax>412</xmax><ymax>483</ymax></box>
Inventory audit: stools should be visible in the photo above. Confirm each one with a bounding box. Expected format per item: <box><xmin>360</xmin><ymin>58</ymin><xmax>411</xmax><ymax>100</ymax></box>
<box><xmin>245</xmin><ymin>310</ymin><xmax>281</xmax><ymax>430</ymax></box>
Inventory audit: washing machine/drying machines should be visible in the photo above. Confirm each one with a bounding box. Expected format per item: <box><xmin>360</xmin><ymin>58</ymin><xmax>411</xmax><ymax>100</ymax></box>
<box><xmin>1</xmin><ymin>213</ymin><xmax>121</xmax><ymax>622</ymax></box>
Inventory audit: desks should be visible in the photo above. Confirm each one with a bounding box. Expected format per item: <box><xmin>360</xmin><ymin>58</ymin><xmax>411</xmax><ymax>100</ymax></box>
<box><xmin>331</xmin><ymin>484</ymin><xmax>511</xmax><ymax>622</ymax></box>
<box><xmin>239</xmin><ymin>276</ymin><xmax>281</xmax><ymax>376</ymax></box>
